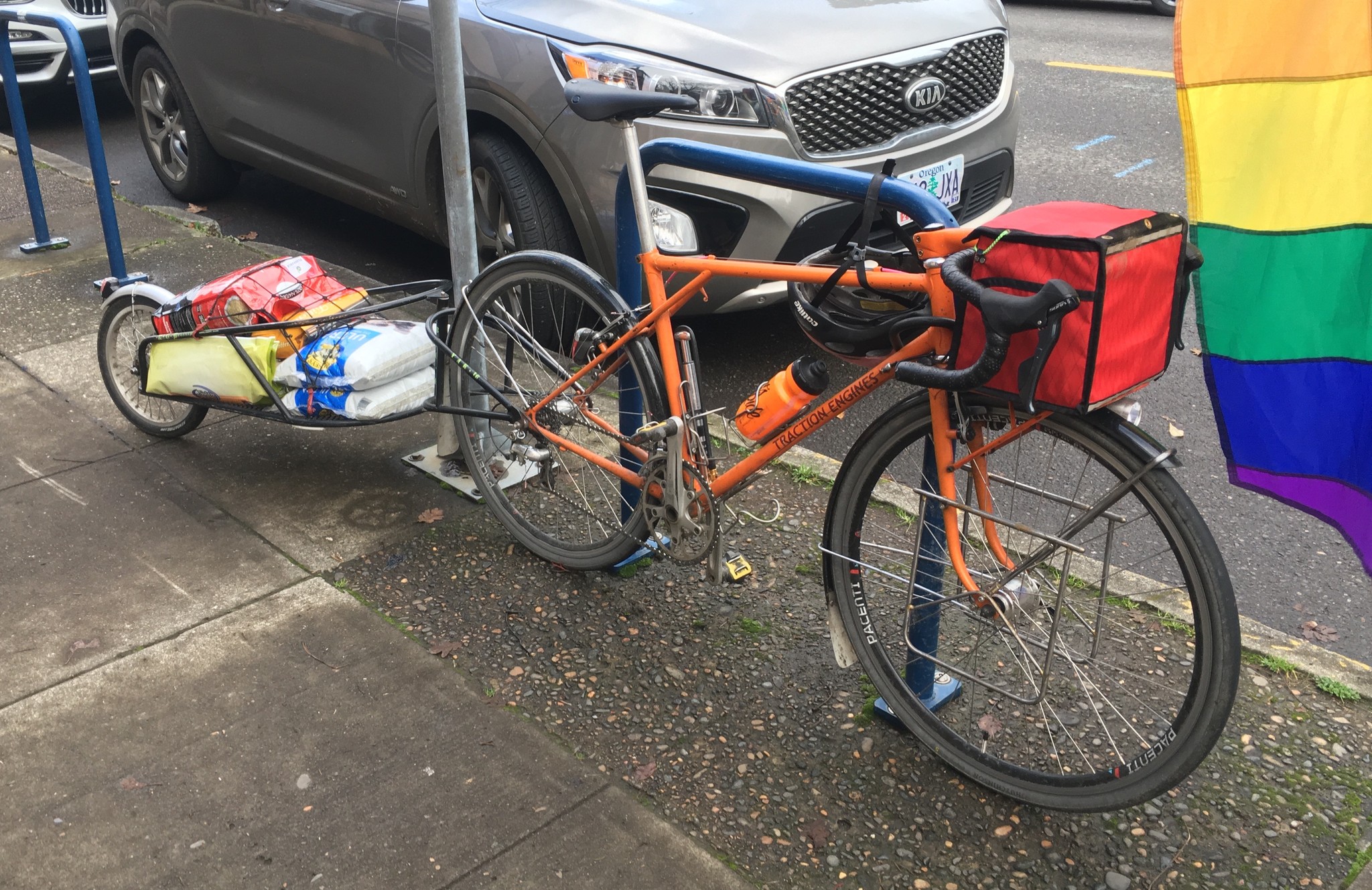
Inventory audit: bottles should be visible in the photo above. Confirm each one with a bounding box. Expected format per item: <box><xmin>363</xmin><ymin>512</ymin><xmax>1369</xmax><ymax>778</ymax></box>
<box><xmin>734</xmin><ymin>355</ymin><xmax>830</xmax><ymax>442</ymax></box>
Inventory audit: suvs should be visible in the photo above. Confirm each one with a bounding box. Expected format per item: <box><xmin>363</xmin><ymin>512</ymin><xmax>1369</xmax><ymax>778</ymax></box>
<box><xmin>113</xmin><ymin>0</ymin><xmax>1016</xmax><ymax>353</ymax></box>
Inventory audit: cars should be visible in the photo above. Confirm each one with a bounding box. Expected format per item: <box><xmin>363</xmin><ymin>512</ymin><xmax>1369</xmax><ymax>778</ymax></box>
<box><xmin>0</xmin><ymin>0</ymin><xmax>135</xmax><ymax>133</ymax></box>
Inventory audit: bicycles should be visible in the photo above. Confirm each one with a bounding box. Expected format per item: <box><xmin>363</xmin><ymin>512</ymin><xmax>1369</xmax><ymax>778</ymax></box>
<box><xmin>425</xmin><ymin>72</ymin><xmax>1245</xmax><ymax>814</ymax></box>
